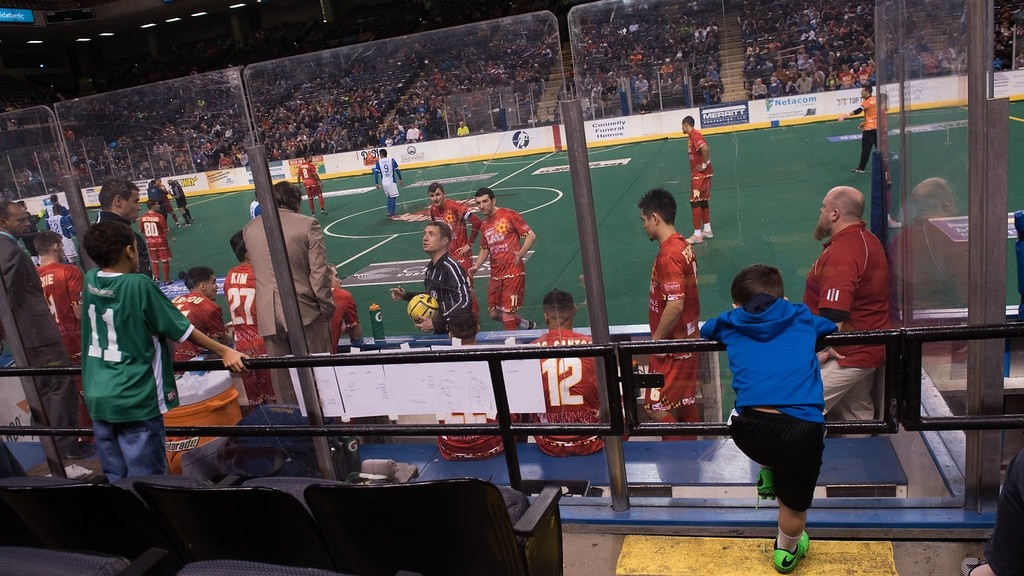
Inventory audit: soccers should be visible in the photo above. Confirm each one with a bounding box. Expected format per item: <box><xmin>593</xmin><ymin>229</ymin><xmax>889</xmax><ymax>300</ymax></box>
<box><xmin>406</xmin><ymin>293</ymin><xmax>440</xmax><ymax>324</ymax></box>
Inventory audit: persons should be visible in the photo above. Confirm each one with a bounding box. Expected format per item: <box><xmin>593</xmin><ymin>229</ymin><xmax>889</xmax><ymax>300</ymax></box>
<box><xmin>552</xmin><ymin>0</ymin><xmax>724</xmax><ymax>124</ymax></box>
<box><xmin>154</xmin><ymin>176</ymin><xmax>182</xmax><ymax>228</ymax></box>
<box><xmin>638</xmin><ymin>187</ymin><xmax>703</xmax><ymax>441</ymax></box>
<box><xmin>838</xmin><ymin>86</ymin><xmax>877</xmax><ymax>173</ymax></box>
<box><xmin>389</xmin><ymin>221</ymin><xmax>480</xmax><ymax>346</ymax></box>
<box><xmin>734</xmin><ymin>0</ymin><xmax>968</xmax><ymax>101</ymax></box>
<box><xmin>147</xmin><ymin>180</ymin><xmax>163</xmax><ymax>213</ymax></box>
<box><xmin>297</xmin><ymin>152</ymin><xmax>328</xmax><ymax>217</ymax></box>
<box><xmin>428</xmin><ymin>182</ymin><xmax>482</xmax><ymax>334</ymax></box>
<box><xmin>167</xmin><ymin>179</ymin><xmax>194</xmax><ymax>228</ymax></box>
<box><xmin>467</xmin><ymin>187</ymin><xmax>538</xmax><ymax>331</ymax></box>
<box><xmin>529</xmin><ymin>288</ymin><xmax>629</xmax><ymax>456</ymax></box>
<box><xmin>700</xmin><ymin>263</ymin><xmax>839</xmax><ymax>574</ymax></box>
<box><xmin>437</xmin><ymin>308</ymin><xmax>529</xmax><ymax>461</ymax></box>
<box><xmin>0</xmin><ymin>0</ymin><xmax>562</xmax><ymax>194</ymax></box>
<box><xmin>374</xmin><ymin>149</ymin><xmax>403</xmax><ymax>219</ymax></box>
<box><xmin>986</xmin><ymin>0</ymin><xmax>1024</xmax><ymax>71</ymax></box>
<box><xmin>0</xmin><ymin>181</ymin><xmax>364</xmax><ymax>485</ymax></box>
<box><xmin>681</xmin><ymin>116</ymin><xmax>714</xmax><ymax>244</ymax></box>
<box><xmin>889</xmin><ymin>179</ymin><xmax>969</xmax><ymax>384</ymax></box>
<box><xmin>960</xmin><ymin>446</ymin><xmax>1024</xmax><ymax>576</ymax></box>
<box><xmin>803</xmin><ymin>185</ymin><xmax>890</xmax><ymax>440</ymax></box>
<box><xmin>138</xmin><ymin>199</ymin><xmax>176</xmax><ymax>286</ymax></box>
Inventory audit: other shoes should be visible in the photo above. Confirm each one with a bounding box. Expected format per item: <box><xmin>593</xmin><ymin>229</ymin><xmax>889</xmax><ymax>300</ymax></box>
<box><xmin>189</xmin><ymin>217</ymin><xmax>194</xmax><ymax>223</ymax></box>
<box><xmin>528</xmin><ymin>320</ymin><xmax>536</xmax><ymax>330</ymax></box>
<box><xmin>387</xmin><ymin>213</ymin><xmax>391</xmax><ymax>219</ymax></box>
<box><xmin>164</xmin><ymin>280</ymin><xmax>174</xmax><ymax>285</ymax></box>
<box><xmin>182</xmin><ymin>223</ymin><xmax>192</xmax><ymax>227</ymax></box>
<box><xmin>960</xmin><ymin>557</ymin><xmax>987</xmax><ymax>576</ymax></box>
<box><xmin>852</xmin><ymin>168</ymin><xmax>865</xmax><ymax>173</ymax></box>
<box><xmin>176</xmin><ymin>223</ymin><xmax>181</xmax><ymax>228</ymax></box>
<box><xmin>154</xmin><ymin>278</ymin><xmax>160</xmax><ymax>284</ymax></box>
<box><xmin>312</xmin><ymin>213</ymin><xmax>316</xmax><ymax>217</ymax></box>
<box><xmin>320</xmin><ymin>209</ymin><xmax>328</xmax><ymax>214</ymax></box>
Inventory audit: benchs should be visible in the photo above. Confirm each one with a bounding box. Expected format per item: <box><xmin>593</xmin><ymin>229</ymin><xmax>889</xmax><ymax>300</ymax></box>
<box><xmin>353</xmin><ymin>442</ymin><xmax>908</xmax><ymax>498</ymax></box>
<box><xmin>0</xmin><ymin>475</ymin><xmax>564</xmax><ymax>576</ymax></box>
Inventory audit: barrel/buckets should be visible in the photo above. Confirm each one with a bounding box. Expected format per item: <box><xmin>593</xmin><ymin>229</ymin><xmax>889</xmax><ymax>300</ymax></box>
<box><xmin>162</xmin><ymin>356</ymin><xmax>244</xmax><ymax>475</ymax></box>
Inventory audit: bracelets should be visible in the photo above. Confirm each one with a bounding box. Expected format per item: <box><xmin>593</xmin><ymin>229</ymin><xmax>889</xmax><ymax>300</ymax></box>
<box><xmin>702</xmin><ymin>163</ymin><xmax>707</xmax><ymax>170</ymax></box>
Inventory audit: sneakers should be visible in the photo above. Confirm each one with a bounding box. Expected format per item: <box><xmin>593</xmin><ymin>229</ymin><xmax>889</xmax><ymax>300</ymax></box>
<box><xmin>685</xmin><ymin>234</ymin><xmax>704</xmax><ymax>244</ymax></box>
<box><xmin>774</xmin><ymin>529</ymin><xmax>811</xmax><ymax>573</ymax></box>
<box><xmin>701</xmin><ymin>230</ymin><xmax>713</xmax><ymax>238</ymax></box>
<box><xmin>757</xmin><ymin>467</ymin><xmax>776</xmax><ymax>501</ymax></box>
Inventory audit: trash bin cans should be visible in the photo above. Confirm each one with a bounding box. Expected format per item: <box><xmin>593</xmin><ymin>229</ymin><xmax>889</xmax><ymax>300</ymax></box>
<box><xmin>158</xmin><ymin>369</ymin><xmax>246</xmax><ymax>476</ymax></box>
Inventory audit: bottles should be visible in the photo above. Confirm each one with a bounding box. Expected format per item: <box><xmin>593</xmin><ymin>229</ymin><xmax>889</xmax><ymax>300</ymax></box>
<box><xmin>369</xmin><ymin>303</ymin><xmax>384</xmax><ymax>339</ymax></box>
